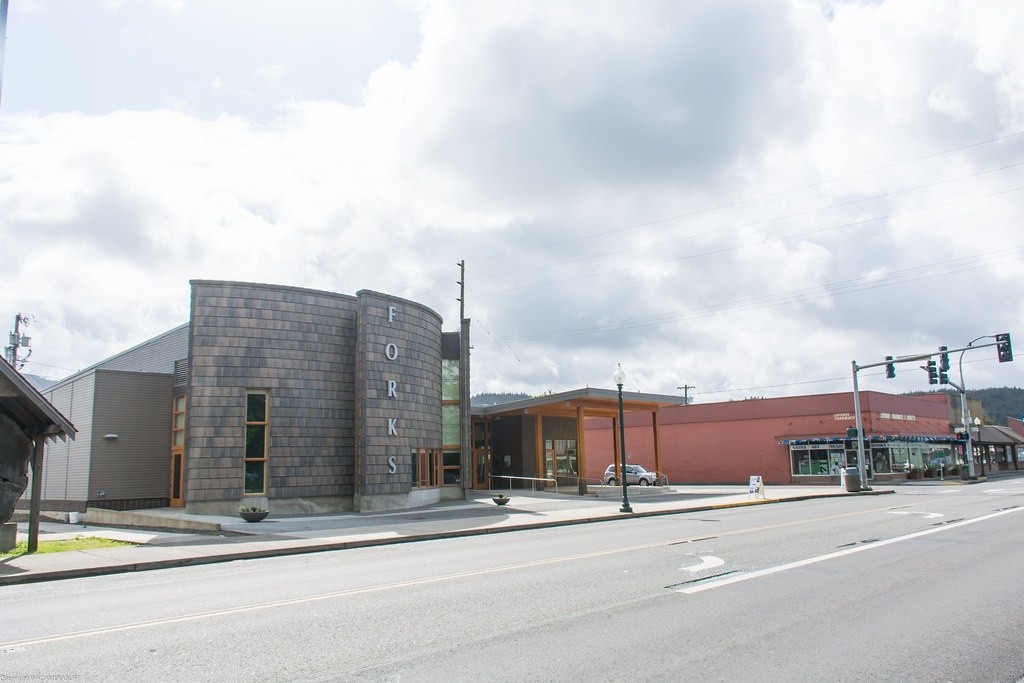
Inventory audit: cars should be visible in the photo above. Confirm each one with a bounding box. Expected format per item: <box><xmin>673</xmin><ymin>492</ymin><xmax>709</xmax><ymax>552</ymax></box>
<box><xmin>977</xmin><ymin>451</ymin><xmax>1005</xmax><ymax>463</ymax></box>
<box><xmin>1017</xmin><ymin>447</ymin><xmax>1024</xmax><ymax>458</ymax></box>
<box><xmin>891</xmin><ymin>460</ymin><xmax>915</xmax><ymax>472</ymax></box>
<box><xmin>799</xmin><ymin>460</ymin><xmax>808</xmax><ymax>466</ymax></box>
<box><xmin>931</xmin><ymin>457</ymin><xmax>952</xmax><ymax>469</ymax></box>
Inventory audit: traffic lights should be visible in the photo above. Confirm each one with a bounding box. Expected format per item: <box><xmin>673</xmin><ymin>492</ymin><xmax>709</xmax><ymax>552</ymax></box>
<box><xmin>939</xmin><ymin>367</ymin><xmax>948</xmax><ymax>384</ymax></box>
<box><xmin>957</xmin><ymin>432</ymin><xmax>968</xmax><ymax>440</ymax></box>
<box><xmin>927</xmin><ymin>360</ymin><xmax>937</xmax><ymax>384</ymax></box>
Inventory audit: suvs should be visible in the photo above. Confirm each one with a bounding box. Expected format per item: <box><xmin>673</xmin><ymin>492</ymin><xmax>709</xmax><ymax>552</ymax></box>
<box><xmin>604</xmin><ymin>463</ymin><xmax>657</xmax><ymax>487</ymax></box>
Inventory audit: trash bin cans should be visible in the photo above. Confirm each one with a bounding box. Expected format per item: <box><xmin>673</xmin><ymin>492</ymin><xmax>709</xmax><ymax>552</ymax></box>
<box><xmin>843</xmin><ymin>474</ymin><xmax>861</xmax><ymax>492</ymax></box>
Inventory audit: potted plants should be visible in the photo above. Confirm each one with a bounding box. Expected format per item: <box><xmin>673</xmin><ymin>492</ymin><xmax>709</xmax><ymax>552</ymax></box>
<box><xmin>238</xmin><ymin>506</ymin><xmax>270</xmax><ymax>523</ymax></box>
<box><xmin>493</xmin><ymin>494</ymin><xmax>510</xmax><ymax>506</ymax></box>
<box><xmin>908</xmin><ymin>468</ymin><xmax>924</xmax><ymax>479</ymax></box>
<box><xmin>927</xmin><ymin>463</ymin><xmax>940</xmax><ymax>478</ymax></box>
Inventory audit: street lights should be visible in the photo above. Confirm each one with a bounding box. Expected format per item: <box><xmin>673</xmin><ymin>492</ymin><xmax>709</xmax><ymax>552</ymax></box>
<box><xmin>614</xmin><ymin>362</ymin><xmax>632</xmax><ymax>513</ymax></box>
<box><xmin>974</xmin><ymin>416</ymin><xmax>985</xmax><ymax>477</ymax></box>
